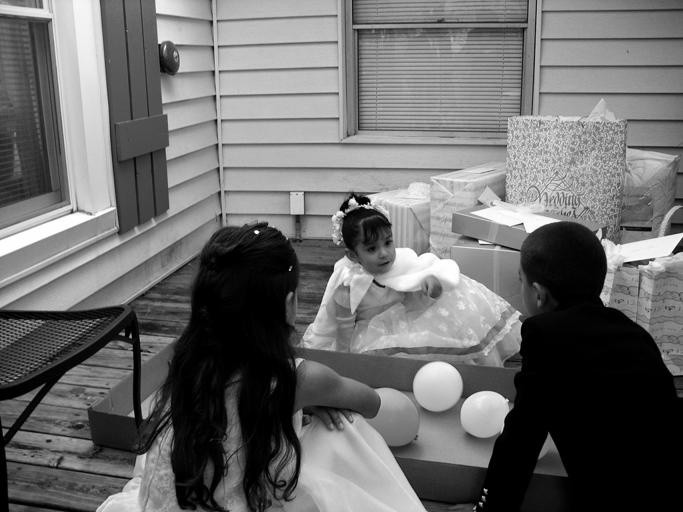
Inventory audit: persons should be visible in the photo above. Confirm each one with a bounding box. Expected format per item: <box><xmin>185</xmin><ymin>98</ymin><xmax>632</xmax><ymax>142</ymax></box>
<box><xmin>297</xmin><ymin>191</ymin><xmax>526</xmax><ymax>369</ymax></box>
<box><xmin>95</xmin><ymin>221</ymin><xmax>425</xmax><ymax>512</ymax></box>
<box><xmin>445</xmin><ymin>221</ymin><xmax>682</xmax><ymax>512</ymax></box>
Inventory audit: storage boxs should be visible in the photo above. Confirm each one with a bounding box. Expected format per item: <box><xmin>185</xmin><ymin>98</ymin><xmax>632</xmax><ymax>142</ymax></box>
<box><xmin>363</xmin><ymin>160</ymin><xmax>564</xmax><ymax>324</ymax></box>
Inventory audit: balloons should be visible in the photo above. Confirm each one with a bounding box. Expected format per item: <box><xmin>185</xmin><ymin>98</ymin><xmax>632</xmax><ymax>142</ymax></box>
<box><xmin>361</xmin><ymin>387</ymin><xmax>420</xmax><ymax>448</ymax></box>
<box><xmin>412</xmin><ymin>361</ymin><xmax>462</xmax><ymax>414</ymax></box>
<box><xmin>538</xmin><ymin>433</ymin><xmax>550</xmax><ymax>460</ymax></box>
<box><xmin>459</xmin><ymin>390</ymin><xmax>510</xmax><ymax>440</ymax></box>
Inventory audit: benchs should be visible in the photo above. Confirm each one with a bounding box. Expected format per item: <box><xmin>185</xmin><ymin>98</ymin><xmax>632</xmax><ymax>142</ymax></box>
<box><xmin>0</xmin><ymin>305</ymin><xmax>141</xmax><ymax>511</ymax></box>
